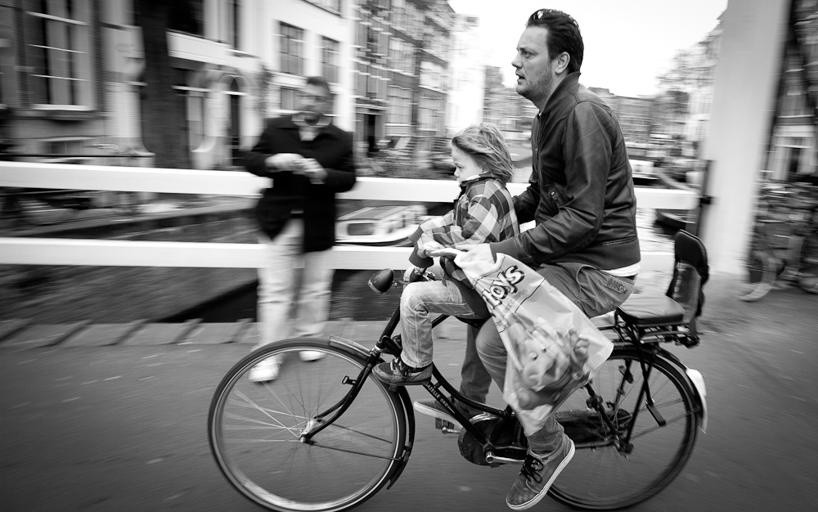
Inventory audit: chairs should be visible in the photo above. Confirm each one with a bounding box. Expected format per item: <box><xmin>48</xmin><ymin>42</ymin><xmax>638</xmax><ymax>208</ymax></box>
<box><xmin>613</xmin><ymin>228</ymin><xmax>710</xmax><ymax>346</ymax></box>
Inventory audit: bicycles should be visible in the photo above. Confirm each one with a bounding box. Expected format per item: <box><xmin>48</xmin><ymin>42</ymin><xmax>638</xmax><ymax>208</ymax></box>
<box><xmin>206</xmin><ymin>227</ymin><xmax>710</xmax><ymax>512</ymax></box>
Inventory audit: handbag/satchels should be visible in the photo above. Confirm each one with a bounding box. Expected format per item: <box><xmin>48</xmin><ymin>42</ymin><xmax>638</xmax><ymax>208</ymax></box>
<box><xmin>453</xmin><ymin>251</ymin><xmax>615</xmax><ymax>438</ymax></box>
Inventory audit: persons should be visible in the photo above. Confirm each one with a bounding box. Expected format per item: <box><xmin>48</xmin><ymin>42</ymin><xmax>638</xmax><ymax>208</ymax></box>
<box><xmin>413</xmin><ymin>9</ymin><xmax>645</xmax><ymax>510</ymax></box>
<box><xmin>239</xmin><ymin>78</ymin><xmax>357</xmax><ymax>384</ymax></box>
<box><xmin>371</xmin><ymin>123</ymin><xmax>522</xmax><ymax>425</ymax></box>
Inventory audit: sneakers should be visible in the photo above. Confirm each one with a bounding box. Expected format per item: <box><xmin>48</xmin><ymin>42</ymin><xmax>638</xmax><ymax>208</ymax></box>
<box><xmin>506</xmin><ymin>433</ymin><xmax>575</xmax><ymax>511</ymax></box>
<box><xmin>249</xmin><ymin>355</ymin><xmax>282</xmax><ymax>382</ymax></box>
<box><xmin>414</xmin><ymin>394</ymin><xmax>487</xmax><ymax>430</ymax></box>
<box><xmin>299</xmin><ymin>351</ymin><xmax>326</xmax><ymax>361</ymax></box>
<box><xmin>372</xmin><ymin>356</ymin><xmax>433</xmax><ymax>386</ymax></box>
